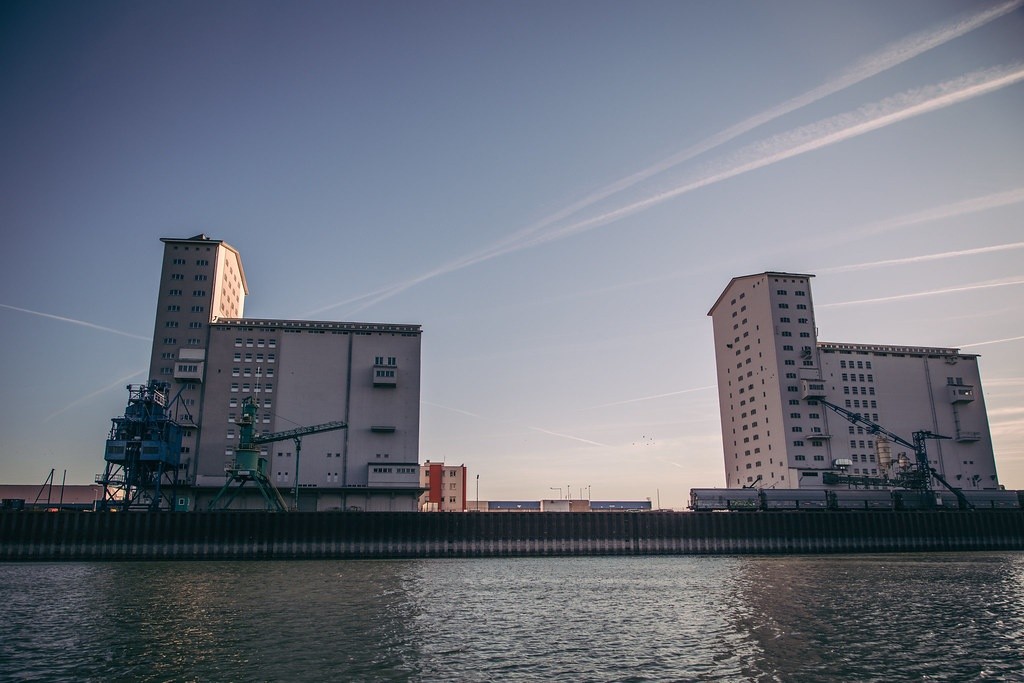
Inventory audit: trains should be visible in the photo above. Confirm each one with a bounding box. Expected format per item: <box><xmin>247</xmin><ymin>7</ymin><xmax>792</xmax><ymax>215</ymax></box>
<box><xmin>685</xmin><ymin>485</ymin><xmax>1023</xmax><ymax>512</ymax></box>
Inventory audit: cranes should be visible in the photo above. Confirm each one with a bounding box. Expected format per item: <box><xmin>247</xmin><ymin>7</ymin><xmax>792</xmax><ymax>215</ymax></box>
<box><xmin>205</xmin><ymin>394</ymin><xmax>350</xmax><ymax>509</ymax></box>
<box><xmin>809</xmin><ymin>394</ymin><xmax>953</xmax><ymax>488</ymax></box>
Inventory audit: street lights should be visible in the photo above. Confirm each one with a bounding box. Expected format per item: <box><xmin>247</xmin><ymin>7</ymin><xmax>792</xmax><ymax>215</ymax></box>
<box><xmin>550</xmin><ymin>487</ymin><xmax>562</xmax><ymax>499</ymax></box>
<box><xmin>476</xmin><ymin>474</ymin><xmax>480</xmax><ymax>510</ymax></box>
